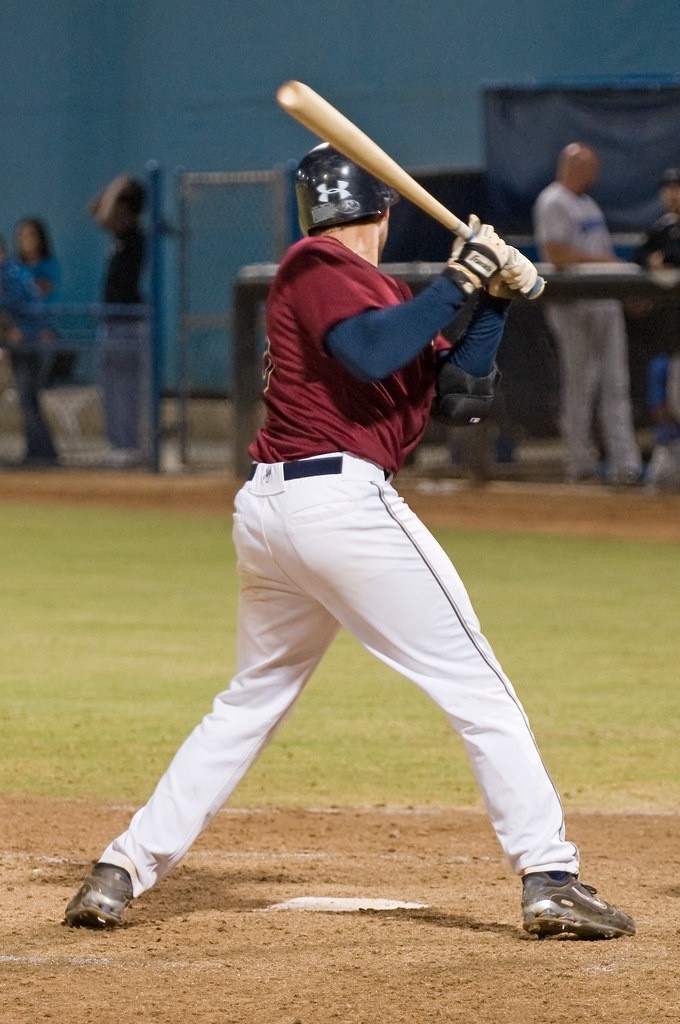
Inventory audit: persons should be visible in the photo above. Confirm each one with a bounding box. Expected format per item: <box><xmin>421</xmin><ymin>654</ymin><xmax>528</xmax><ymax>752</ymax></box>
<box><xmin>1</xmin><ymin>173</ymin><xmax>150</xmax><ymax>471</ymax></box>
<box><xmin>66</xmin><ymin>141</ymin><xmax>635</xmax><ymax>938</ymax></box>
<box><xmin>532</xmin><ymin>144</ymin><xmax>679</xmax><ymax>485</ymax></box>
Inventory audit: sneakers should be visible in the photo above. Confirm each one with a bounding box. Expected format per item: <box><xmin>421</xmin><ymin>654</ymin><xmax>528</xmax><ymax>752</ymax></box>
<box><xmin>520</xmin><ymin>872</ymin><xmax>635</xmax><ymax>941</ymax></box>
<box><xmin>64</xmin><ymin>864</ymin><xmax>133</xmax><ymax>926</ymax></box>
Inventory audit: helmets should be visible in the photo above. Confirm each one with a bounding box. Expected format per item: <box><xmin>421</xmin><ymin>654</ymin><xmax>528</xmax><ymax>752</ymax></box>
<box><xmin>294</xmin><ymin>141</ymin><xmax>401</xmax><ymax>238</ymax></box>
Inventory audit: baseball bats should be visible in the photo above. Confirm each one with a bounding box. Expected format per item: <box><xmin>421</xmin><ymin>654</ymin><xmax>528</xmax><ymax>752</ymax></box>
<box><xmin>276</xmin><ymin>78</ymin><xmax>551</xmax><ymax>304</ymax></box>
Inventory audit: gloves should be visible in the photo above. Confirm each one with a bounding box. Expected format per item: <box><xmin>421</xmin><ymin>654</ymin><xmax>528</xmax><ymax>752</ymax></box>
<box><xmin>487</xmin><ymin>245</ymin><xmax>538</xmax><ymax>299</ymax></box>
<box><xmin>441</xmin><ymin>213</ymin><xmax>509</xmax><ymax>294</ymax></box>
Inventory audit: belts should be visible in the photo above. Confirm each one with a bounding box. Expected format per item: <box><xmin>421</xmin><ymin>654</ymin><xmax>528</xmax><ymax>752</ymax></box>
<box><xmin>245</xmin><ymin>460</ymin><xmax>390</xmax><ymax>482</ymax></box>
